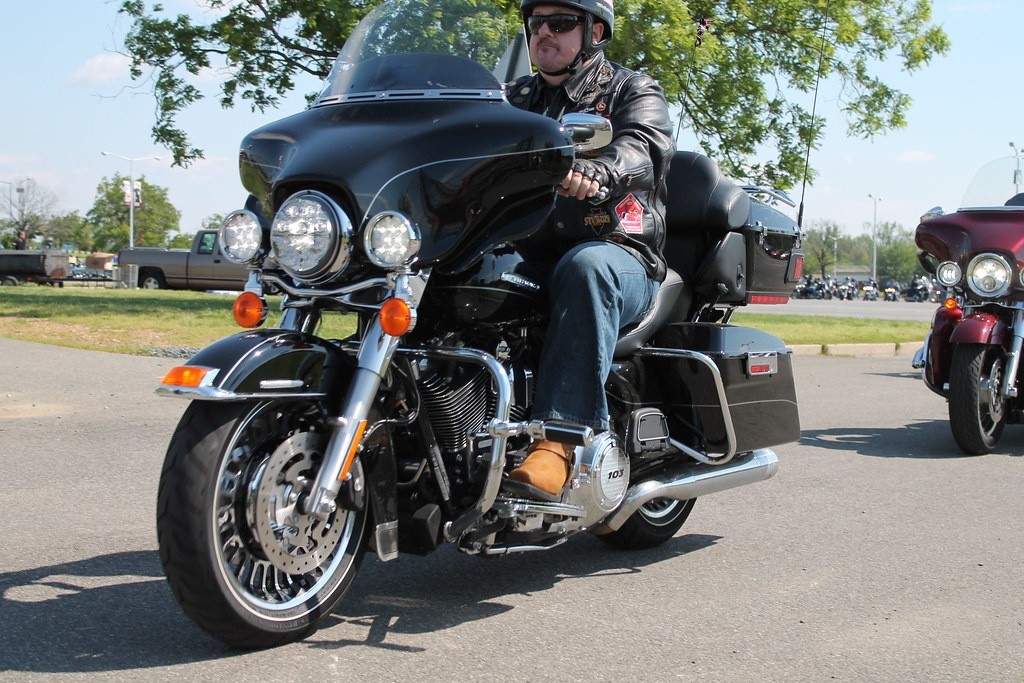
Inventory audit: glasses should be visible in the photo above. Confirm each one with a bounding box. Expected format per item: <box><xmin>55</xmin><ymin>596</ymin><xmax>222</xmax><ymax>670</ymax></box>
<box><xmin>526</xmin><ymin>12</ymin><xmax>597</xmax><ymax>33</ymax></box>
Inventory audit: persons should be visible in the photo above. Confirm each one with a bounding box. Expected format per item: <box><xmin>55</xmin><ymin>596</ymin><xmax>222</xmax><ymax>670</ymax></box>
<box><xmin>804</xmin><ymin>272</ymin><xmax>924</xmax><ymax>302</ymax></box>
<box><xmin>15</xmin><ymin>232</ymin><xmax>26</xmax><ymax>250</ymax></box>
<box><xmin>503</xmin><ymin>1</ymin><xmax>677</xmax><ymax>504</ymax></box>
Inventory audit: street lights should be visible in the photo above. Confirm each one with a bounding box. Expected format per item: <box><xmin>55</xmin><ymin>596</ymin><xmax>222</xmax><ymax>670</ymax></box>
<box><xmin>868</xmin><ymin>193</ymin><xmax>884</xmax><ymax>285</ymax></box>
<box><xmin>1009</xmin><ymin>141</ymin><xmax>1024</xmax><ymax>193</ymax></box>
<box><xmin>101</xmin><ymin>151</ymin><xmax>162</xmax><ymax>250</ymax></box>
<box><xmin>0</xmin><ymin>178</ymin><xmax>29</xmax><ymax>220</ymax></box>
<box><xmin>828</xmin><ymin>234</ymin><xmax>842</xmax><ymax>278</ymax></box>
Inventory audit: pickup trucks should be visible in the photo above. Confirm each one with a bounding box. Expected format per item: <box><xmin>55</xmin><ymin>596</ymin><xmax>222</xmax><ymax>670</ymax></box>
<box><xmin>117</xmin><ymin>228</ymin><xmax>283</xmax><ymax>294</ymax></box>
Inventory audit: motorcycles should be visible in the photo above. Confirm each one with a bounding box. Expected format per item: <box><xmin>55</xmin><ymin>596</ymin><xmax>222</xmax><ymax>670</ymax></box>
<box><xmin>863</xmin><ymin>288</ymin><xmax>878</xmax><ymax>302</ymax></box>
<box><xmin>152</xmin><ymin>0</ymin><xmax>807</xmax><ymax>652</ymax></box>
<box><xmin>884</xmin><ymin>289</ymin><xmax>897</xmax><ymax>302</ymax></box>
<box><xmin>791</xmin><ymin>282</ymin><xmax>858</xmax><ymax>302</ymax></box>
<box><xmin>911</xmin><ymin>192</ymin><xmax>1024</xmax><ymax>456</ymax></box>
<box><xmin>902</xmin><ymin>289</ymin><xmax>930</xmax><ymax>302</ymax></box>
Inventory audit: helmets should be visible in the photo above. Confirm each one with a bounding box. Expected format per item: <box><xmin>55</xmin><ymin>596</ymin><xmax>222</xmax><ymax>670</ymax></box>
<box><xmin>520</xmin><ymin>0</ymin><xmax>615</xmax><ymax>53</ymax></box>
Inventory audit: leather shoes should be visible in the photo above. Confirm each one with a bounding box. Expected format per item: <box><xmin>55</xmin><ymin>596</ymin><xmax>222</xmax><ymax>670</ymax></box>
<box><xmin>501</xmin><ymin>436</ymin><xmax>577</xmax><ymax>504</ymax></box>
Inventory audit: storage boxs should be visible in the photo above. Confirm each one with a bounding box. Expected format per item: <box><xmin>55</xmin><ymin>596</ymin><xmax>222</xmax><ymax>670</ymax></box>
<box><xmin>647</xmin><ymin>321</ymin><xmax>802</xmax><ymax>454</ymax></box>
<box><xmin>744</xmin><ymin>202</ymin><xmax>803</xmax><ymax>304</ymax></box>
